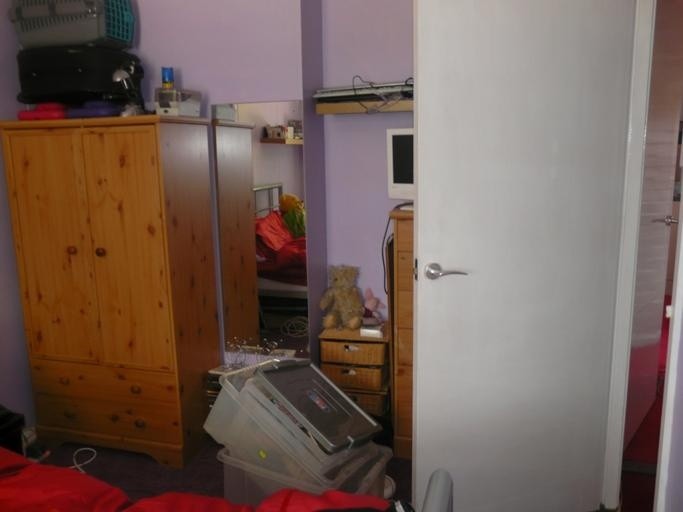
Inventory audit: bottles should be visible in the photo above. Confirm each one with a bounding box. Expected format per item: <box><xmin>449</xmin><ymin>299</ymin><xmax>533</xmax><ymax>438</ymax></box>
<box><xmin>161</xmin><ymin>65</ymin><xmax>174</xmax><ymax>89</ymax></box>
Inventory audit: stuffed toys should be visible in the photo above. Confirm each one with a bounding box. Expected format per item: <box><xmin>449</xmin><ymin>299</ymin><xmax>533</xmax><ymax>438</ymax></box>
<box><xmin>318</xmin><ymin>263</ymin><xmax>364</xmax><ymax>330</ymax></box>
<box><xmin>278</xmin><ymin>192</ymin><xmax>306</xmax><ymax>217</ymax></box>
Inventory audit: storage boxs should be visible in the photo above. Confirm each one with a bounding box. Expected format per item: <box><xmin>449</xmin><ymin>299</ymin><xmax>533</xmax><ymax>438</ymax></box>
<box><xmin>197</xmin><ymin>353</ymin><xmax>397</xmax><ymax>506</ymax></box>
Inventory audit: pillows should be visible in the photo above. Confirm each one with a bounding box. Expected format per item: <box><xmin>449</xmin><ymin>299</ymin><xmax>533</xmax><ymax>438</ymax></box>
<box><xmin>279</xmin><ymin>191</ymin><xmax>306</xmax><ymax>242</ymax></box>
<box><xmin>254</xmin><ymin>209</ymin><xmax>292</xmax><ymax>253</ymax></box>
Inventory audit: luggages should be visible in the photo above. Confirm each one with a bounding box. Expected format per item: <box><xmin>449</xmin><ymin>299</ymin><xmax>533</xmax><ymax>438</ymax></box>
<box><xmin>15</xmin><ymin>46</ymin><xmax>144</xmax><ymax>104</ymax></box>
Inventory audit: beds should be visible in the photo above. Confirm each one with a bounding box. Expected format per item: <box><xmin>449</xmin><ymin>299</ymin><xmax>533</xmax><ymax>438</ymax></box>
<box><xmin>247</xmin><ymin>178</ymin><xmax>307</xmax><ymax>313</ymax></box>
<box><xmin>0</xmin><ymin>440</ymin><xmax>461</xmax><ymax>512</ymax></box>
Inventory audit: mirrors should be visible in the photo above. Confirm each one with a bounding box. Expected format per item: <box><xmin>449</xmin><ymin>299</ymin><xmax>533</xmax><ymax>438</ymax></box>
<box><xmin>208</xmin><ymin>99</ymin><xmax>309</xmax><ymax>365</ymax></box>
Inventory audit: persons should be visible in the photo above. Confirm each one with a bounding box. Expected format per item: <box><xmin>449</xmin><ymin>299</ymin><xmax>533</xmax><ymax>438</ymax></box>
<box><xmin>361</xmin><ymin>287</ymin><xmax>385</xmax><ymax>326</ymax></box>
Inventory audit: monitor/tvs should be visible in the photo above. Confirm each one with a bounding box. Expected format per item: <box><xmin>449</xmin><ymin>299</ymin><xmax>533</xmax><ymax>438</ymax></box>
<box><xmin>386</xmin><ymin>128</ymin><xmax>413</xmax><ymax>211</ymax></box>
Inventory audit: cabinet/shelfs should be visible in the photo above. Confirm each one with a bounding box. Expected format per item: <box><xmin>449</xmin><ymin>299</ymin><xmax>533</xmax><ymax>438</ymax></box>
<box><xmin>211</xmin><ymin>118</ymin><xmax>263</xmax><ymax>351</ymax></box>
<box><xmin>387</xmin><ymin>204</ymin><xmax>416</xmax><ymax>463</ymax></box>
<box><xmin>317</xmin><ymin>322</ymin><xmax>389</xmax><ymax>427</ymax></box>
<box><xmin>1</xmin><ymin>114</ymin><xmax>223</xmax><ymax>473</ymax></box>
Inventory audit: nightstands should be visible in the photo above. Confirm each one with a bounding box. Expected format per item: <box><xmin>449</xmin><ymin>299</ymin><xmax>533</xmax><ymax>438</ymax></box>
<box><xmin>1</xmin><ymin>404</ymin><xmax>28</xmax><ymax>457</ymax></box>
